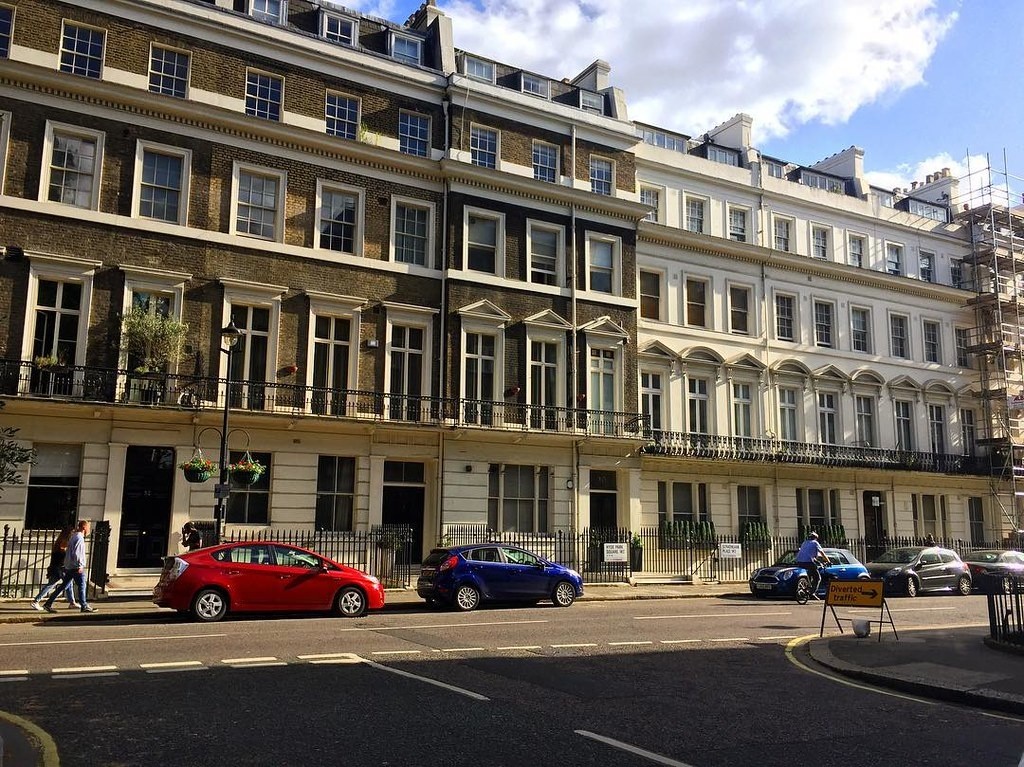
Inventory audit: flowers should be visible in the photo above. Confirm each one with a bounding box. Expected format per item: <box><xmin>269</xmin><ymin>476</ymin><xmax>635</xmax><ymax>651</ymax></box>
<box><xmin>226</xmin><ymin>459</ymin><xmax>267</xmax><ymax>475</ymax></box>
<box><xmin>178</xmin><ymin>458</ymin><xmax>220</xmax><ymax>475</ymax></box>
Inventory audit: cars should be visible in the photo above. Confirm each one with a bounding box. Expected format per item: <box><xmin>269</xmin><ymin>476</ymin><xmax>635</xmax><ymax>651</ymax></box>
<box><xmin>417</xmin><ymin>542</ymin><xmax>586</xmax><ymax>612</ymax></box>
<box><xmin>962</xmin><ymin>550</ymin><xmax>1024</xmax><ymax>595</ymax></box>
<box><xmin>151</xmin><ymin>541</ymin><xmax>386</xmax><ymax>622</ymax></box>
<box><xmin>749</xmin><ymin>548</ymin><xmax>873</xmax><ymax>599</ymax></box>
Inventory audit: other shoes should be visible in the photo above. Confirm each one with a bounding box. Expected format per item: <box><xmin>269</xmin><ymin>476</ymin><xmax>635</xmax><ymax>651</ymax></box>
<box><xmin>810</xmin><ymin>593</ymin><xmax>820</xmax><ymax>602</ymax></box>
<box><xmin>80</xmin><ymin>605</ymin><xmax>98</xmax><ymax>613</ymax></box>
<box><xmin>68</xmin><ymin>603</ymin><xmax>82</xmax><ymax>610</ymax></box>
<box><xmin>43</xmin><ymin>604</ymin><xmax>57</xmax><ymax>613</ymax></box>
<box><xmin>30</xmin><ymin>601</ymin><xmax>43</xmax><ymax>611</ymax></box>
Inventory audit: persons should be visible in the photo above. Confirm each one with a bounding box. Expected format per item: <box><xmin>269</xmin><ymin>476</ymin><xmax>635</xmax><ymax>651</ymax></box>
<box><xmin>31</xmin><ymin>525</ymin><xmax>81</xmax><ymax>609</ymax></box>
<box><xmin>42</xmin><ymin>520</ymin><xmax>98</xmax><ymax>613</ymax></box>
<box><xmin>181</xmin><ymin>522</ymin><xmax>203</xmax><ymax>551</ymax></box>
<box><xmin>797</xmin><ymin>531</ymin><xmax>830</xmax><ymax>600</ymax></box>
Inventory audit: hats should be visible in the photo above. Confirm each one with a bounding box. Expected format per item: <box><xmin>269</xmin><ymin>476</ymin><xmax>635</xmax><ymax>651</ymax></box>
<box><xmin>809</xmin><ymin>531</ymin><xmax>819</xmax><ymax>537</ymax></box>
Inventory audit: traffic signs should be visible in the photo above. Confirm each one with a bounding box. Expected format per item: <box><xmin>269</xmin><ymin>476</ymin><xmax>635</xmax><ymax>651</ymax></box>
<box><xmin>827</xmin><ymin>580</ymin><xmax>886</xmax><ymax>607</ymax></box>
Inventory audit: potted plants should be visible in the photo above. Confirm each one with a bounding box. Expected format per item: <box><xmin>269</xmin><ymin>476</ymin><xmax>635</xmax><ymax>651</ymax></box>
<box><xmin>376</xmin><ymin>531</ymin><xmax>402</xmax><ymax>577</ymax></box>
<box><xmin>112</xmin><ymin>304</ymin><xmax>188</xmax><ymax>409</ymax></box>
<box><xmin>590</xmin><ymin>530</ymin><xmax>601</xmax><ymax>572</ymax></box>
<box><xmin>630</xmin><ymin>532</ymin><xmax>643</xmax><ymax>572</ymax></box>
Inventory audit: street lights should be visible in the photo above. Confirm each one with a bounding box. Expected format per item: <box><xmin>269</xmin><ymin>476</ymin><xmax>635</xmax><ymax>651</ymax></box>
<box><xmin>184</xmin><ymin>314</ymin><xmax>264</xmax><ymax>546</ymax></box>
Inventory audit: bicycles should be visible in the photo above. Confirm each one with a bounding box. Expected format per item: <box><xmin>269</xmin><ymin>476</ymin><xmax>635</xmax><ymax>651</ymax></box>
<box><xmin>794</xmin><ymin>562</ymin><xmax>833</xmax><ymax>605</ymax></box>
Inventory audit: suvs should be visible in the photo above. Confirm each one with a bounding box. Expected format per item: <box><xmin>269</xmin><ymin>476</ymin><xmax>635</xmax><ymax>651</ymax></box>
<box><xmin>864</xmin><ymin>547</ymin><xmax>972</xmax><ymax>597</ymax></box>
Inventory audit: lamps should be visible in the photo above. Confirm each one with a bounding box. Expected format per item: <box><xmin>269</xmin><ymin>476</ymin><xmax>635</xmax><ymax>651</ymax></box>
<box><xmin>511</xmin><ymin>386</ymin><xmax>520</xmax><ymax>395</ymax></box>
<box><xmin>577</xmin><ymin>394</ymin><xmax>585</xmax><ymax>401</ymax></box>
